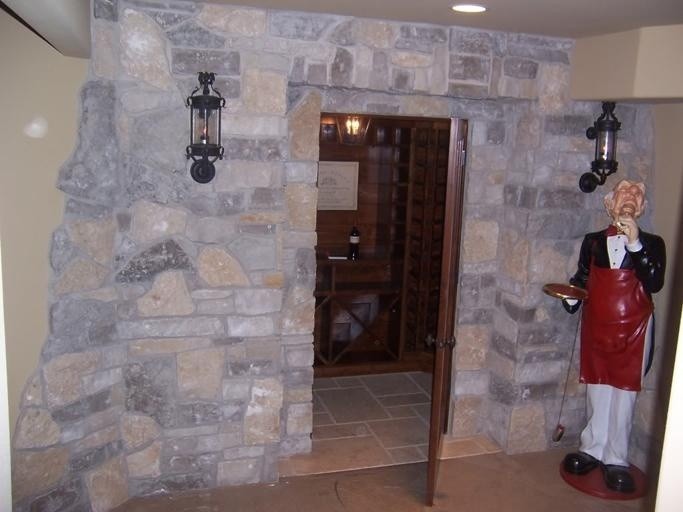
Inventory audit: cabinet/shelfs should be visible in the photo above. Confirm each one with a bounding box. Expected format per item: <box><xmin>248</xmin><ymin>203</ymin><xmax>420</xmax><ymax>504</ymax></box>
<box><xmin>312</xmin><ymin>258</ymin><xmax>406</xmax><ymax>378</ymax></box>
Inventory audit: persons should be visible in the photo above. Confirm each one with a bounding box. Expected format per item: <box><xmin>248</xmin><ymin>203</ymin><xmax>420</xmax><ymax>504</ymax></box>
<box><xmin>561</xmin><ymin>176</ymin><xmax>667</xmax><ymax>492</ymax></box>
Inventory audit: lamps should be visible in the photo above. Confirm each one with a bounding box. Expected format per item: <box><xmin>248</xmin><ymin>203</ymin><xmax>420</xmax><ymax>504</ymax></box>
<box><xmin>343</xmin><ymin>115</ymin><xmax>363</xmax><ymax>144</ymax></box>
<box><xmin>579</xmin><ymin>100</ymin><xmax>622</xmax><ymax>193</ymax></box>
<box><xmin>184</xmin><ymin>71</ymin><xmax>227</xmax><ymax>184</ymax></box>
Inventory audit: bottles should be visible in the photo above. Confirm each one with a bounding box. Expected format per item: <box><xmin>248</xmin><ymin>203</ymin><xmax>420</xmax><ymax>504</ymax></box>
<box><xmin>349</xmin><ymin>220</ymin><xmax>360</xmax><ymax>260</ymax></box>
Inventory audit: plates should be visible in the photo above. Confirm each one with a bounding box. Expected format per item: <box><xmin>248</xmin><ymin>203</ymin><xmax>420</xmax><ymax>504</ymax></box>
<box><xmin>543</xmin><ymin>283</ymin><xmax>588</xmax><ymax>299</ymax></box>
